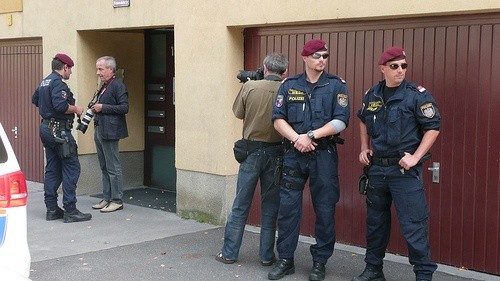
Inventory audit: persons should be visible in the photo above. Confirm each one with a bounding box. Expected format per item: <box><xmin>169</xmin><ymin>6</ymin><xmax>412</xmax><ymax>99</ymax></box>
<box><xmin>267</xmin><ymin>40</ymin><xmax>350</xmax><ymax>281</ymax></box>
<box><xmin>352</xmin><ymin>49</ymin><xmax>441</xmax><ymax>281</ymax></box>
<box><xmin>32</xmin><ymin>54</ymin><xmax>92</xmax><ymax>223</ymax></box>
<box><xmin>92</xmin><ymin>56</ymin><xmax>128</xmax><ymax>213</ymax></box>
<box><xmin>216</xmin><ymin>53</ymin><xmax>288</xmax><ymax>267</ymax></box>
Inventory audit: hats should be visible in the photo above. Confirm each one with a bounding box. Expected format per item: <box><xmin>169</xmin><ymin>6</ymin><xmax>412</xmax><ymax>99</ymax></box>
<box><xmin>378</xmin><ymin>46</ymin><xmax>406</xmax><ymax>65</ymax></box>
<box><xmin>54</xmin><ymin>53</ymin><xmax>74</xmax><ymax>67</ymax></box>
<box><xmin>301</xmin><ymin>40</ymin><xmax>327</xmax><ymax>56</ymax></box>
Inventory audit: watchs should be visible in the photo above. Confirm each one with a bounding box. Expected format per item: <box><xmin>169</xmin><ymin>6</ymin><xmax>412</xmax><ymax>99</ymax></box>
<box><xmin>307</xmin><ymin>131</ymin><xmax>316</xmax><ymax>142</ymax></box>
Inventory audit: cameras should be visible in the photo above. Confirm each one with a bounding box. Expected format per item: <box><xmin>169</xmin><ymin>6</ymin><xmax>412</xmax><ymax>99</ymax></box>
<box><xmin>236</xmin><ymin>66</ymin><xmax>264</xmax><ymax>84</ymax></box>
<box><xmin>76</xmin><ymin>101</ymin><xmax>95</xmax><ymax>135</ymax></box>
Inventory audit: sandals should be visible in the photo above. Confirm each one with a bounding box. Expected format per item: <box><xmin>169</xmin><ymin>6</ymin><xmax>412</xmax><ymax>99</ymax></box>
<box><xmin>261</xmin><ymin>256</ymin><xmax>276</xmax><ymax>266</ymax></box>
<box><xmin>215</xmin><ymin>251</ymin><xmax>236</xmax><ymax>263</ymax></box>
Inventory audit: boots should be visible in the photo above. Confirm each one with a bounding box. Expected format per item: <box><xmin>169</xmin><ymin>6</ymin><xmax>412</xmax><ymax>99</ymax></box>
<box><xmin>268</xmin><ymin>258</ymin><xmax>296</xmax><ymax>279</ymax></box>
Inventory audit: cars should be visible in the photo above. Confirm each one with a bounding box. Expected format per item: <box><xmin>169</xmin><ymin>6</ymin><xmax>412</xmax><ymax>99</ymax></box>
<box><xmin>0</xmin><ymin>124</ymin><xmax>34</xmax><ymax>281</ymax></box>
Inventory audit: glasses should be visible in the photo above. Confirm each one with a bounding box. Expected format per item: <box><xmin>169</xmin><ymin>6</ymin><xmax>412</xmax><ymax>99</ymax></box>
<box><xmin>312</xmin><ymin>52</ymin><xmax>329</xmax><ymax>59</ymax></box>
<box><xmin>386</xmin><ymin>63</ymin><xmax>408</xmax><ymax>69</ymax></box>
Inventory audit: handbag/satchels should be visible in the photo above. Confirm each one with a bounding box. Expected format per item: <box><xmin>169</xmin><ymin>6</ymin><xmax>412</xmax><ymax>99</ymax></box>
<box><xmin>232</xmin><ymin>138</ymin><xmax>282</xmax><ymax>163</ymax></box>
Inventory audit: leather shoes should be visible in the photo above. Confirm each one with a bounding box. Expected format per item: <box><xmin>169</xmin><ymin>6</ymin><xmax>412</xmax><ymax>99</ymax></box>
<box><xmin>63</xmin><ymin>208</ymin><xmax>92</xmax><ymax>223</ymax></box>
<box><xmin>46</xmin><ymin>207</ymin><xmax>64</xmax><ymax>220</ymax></box>
<box><xmin>350</xmin><ymin>263</ymin><xmax>386</xmax><ymax>281</ymax></box>
<box><xmin>309</xmin><ymin>260</ymin><xmax>326</xmax><ymax>280</ymax></box>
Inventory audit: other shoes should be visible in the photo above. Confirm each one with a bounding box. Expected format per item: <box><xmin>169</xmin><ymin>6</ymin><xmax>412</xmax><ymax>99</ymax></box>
<box><xmin>92</xmin><ymin>199</ymin><xmax>112</xmax><ymax>209</ymax></box>
<box><xmin>100</xmin><ymin>202</ymin><xmax>124</xmax><ymax>212</ymax></box>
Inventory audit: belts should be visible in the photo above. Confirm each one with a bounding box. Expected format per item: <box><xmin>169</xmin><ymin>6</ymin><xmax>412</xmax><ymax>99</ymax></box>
<box><xmin>370</xmin><ymin>157</ymin><xmax>400</xmax><ymax>165</ymax></box>
